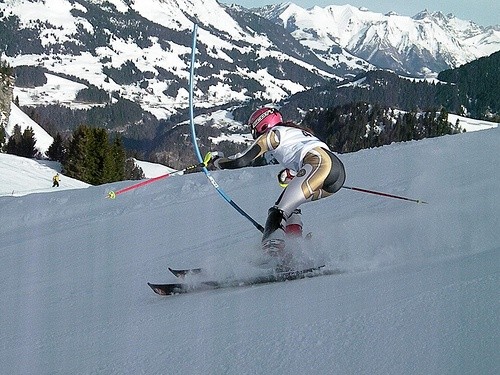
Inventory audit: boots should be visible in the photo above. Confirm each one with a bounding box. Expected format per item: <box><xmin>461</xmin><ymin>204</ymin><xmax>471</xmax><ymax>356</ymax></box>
<box><xmin>255</xmin><ymin>239</ymin><xmax>288</xmax><ymax>264</ymax></box>
<box><xmin>284</xmin><ymin>239</ymin><xmax>310</xmax><ymax>265</ymax></box>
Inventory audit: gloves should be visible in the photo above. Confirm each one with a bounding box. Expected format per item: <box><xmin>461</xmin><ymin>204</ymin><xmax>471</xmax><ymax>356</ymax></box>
<box><xmin>204</xmin><ymin>150</ymin><xmax>224</xmax><ymax>171</ymax></box>
<box><xmin>279</xmin><ymin>169</ymin><xmax>296</xmax><ymax>186</ymax></box>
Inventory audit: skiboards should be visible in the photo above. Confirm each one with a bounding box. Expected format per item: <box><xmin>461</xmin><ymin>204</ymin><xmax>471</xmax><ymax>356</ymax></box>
<box><xmin>147</xmin><ymin>264</ymin><xmax>325</xmax><ymax>296</ymax></box>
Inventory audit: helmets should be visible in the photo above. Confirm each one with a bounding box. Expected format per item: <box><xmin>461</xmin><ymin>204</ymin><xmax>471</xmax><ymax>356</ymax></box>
<box><xmin>249</xmin><ymin>107</ymin><xmax>282</xmax><ymax>133</ymax></box>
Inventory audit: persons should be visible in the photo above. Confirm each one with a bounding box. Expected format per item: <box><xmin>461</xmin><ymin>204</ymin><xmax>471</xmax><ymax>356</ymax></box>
<box><xmin>207</xmin><ymin>107</ymin><xmax>348</xmax><ymax>266</ymax></box>
<box><xmin>52</xmin><ymin>173</ymin><xmax>62</xmax><ymax>187</ymax></box>
